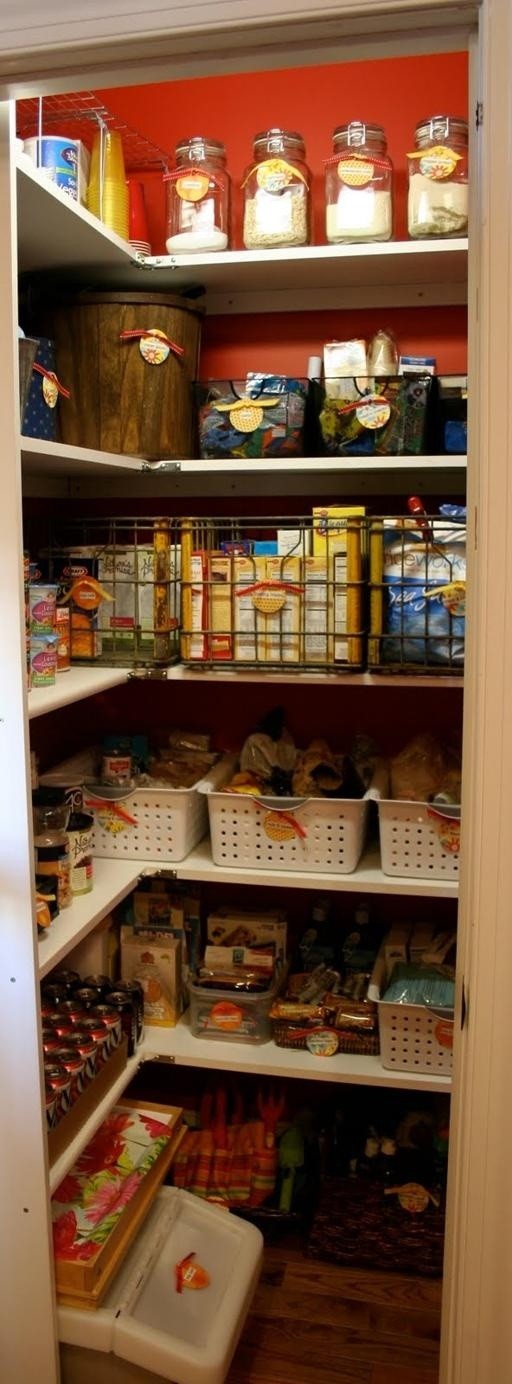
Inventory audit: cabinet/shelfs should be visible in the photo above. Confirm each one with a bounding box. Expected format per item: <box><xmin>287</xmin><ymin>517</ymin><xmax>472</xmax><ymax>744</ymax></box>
<box><xmin>0</xmin><ymin>134</ymin><xmax>511</xmax><ymax>1384</ymax></box>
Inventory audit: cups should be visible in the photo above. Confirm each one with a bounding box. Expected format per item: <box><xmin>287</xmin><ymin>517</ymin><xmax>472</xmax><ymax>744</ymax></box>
<box><xmin>88</xmin><ymin>130</ymin><xmax>129</xmax><ymax>244</ymax></box>
<box><xmin>125</xmin><ymin>179</ymin><xmax>152</xmax><ymax>256</ymax></box>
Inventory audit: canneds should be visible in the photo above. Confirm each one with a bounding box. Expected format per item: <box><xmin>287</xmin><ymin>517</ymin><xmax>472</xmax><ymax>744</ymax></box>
<box><xmin>112</xmin><ymin>976</ymin><xmax>145</xmax><ymax>1039</ymax></box>
<box><xmin>61</xmin><ymin>1032</ymin><xmax>100</xmax><ymax>1088</ymax></box>
<box><xmin>43</xmin><ymin>1064</ymin><xmax>74</xmax><ymax>1117</ymax></box>
<box><xmin>63</xmin><ymin>813</ymin><xmax>95</xmax><ymax>894</ymax></box>
<box><xmin>57</xmin><ymin>999</ymin><xmax>88</xmax><ymax>1023</ymax></box>
<box><xmin>42</xmin><ymin>1031</ymin><xmax>59</xmax><ymax>1056</ymax></box>
<box><xmin>51</xmin><ymin>967</ymin><xmax>84</xmax><ymax>988</ymax></box>
<box><xmin>32</xmin><ymin>784</ymin><xmax>67</xmax><ymax>832</ymax></box>
<box><xmin>103</xmin><ymin>991</ymin><xmax>137</xmax><ymax>1059</ymax></box>
<box><xmin>72</xmin><ymin>986</ymin><xmax>100</xmax><ymax>1007</ymax></box>
<box><xmin>38</xmin><ymin>771</ymin><xmax>86</xmax><ymax>815</ymax></box>
<box><xmin>97</xmin><ymin>749</ymin><xmax>133</xmax><ymax>792</ymax></box>
<box><xmin>76</xmin><ymin>1018</ymin><xmax>114</xmax><ymax>1070</ymax></box>
<box><xmin>404</xmin><ymin>114</ymin><xmax>468</xmax><ymax>238</ymax></box>
<box><xmin>161</xmin><ymin>133</ymin><xmax>233</xmax><ymax>256</ymax></box>
<box><xmin>82</xmin><ymin>972</ymin><xmax>113</xmax><ymax>992</ymax></box>
<box><xmin>91</xmin><ymin>1002</ymin><xmax>124</xmax><ymax>1049</ymax></box>
<box><xmin>39</xmin><ymin>980</ymin><xmax>68</xmax><ymax>999</ymax></box>
<box><xmin>321</xmin><ymin>120</ymin><xmax>395</xmax><ymax>246</ymax></box>
<box><xmin>44</xmin><ymin>1085</ymin><xmax>54</xmax><ymax>1130</ymax></box>
<box><xmin>240</xmin><ymin>126</ymin><xmax>314</xmax><ymax>250</ymax></box>
<box><xmin>40</xmin><ymin>996</ymin><xmax>55</xmax><ymax>1015</ymax></box>
<box><xmin>41</xmin><ymin>1017</ymin><xmax>58</xmax><ymax>1039</ymax></box>
<box><xmin>48</xmin><ymin>1045</ymin><xmax>85</xmax><ymax>1103</ymax></box>
<box><xmin>34</xmin><ymin>832</ymin><xmax>69</xmax><ymax>909</ymax></box>
